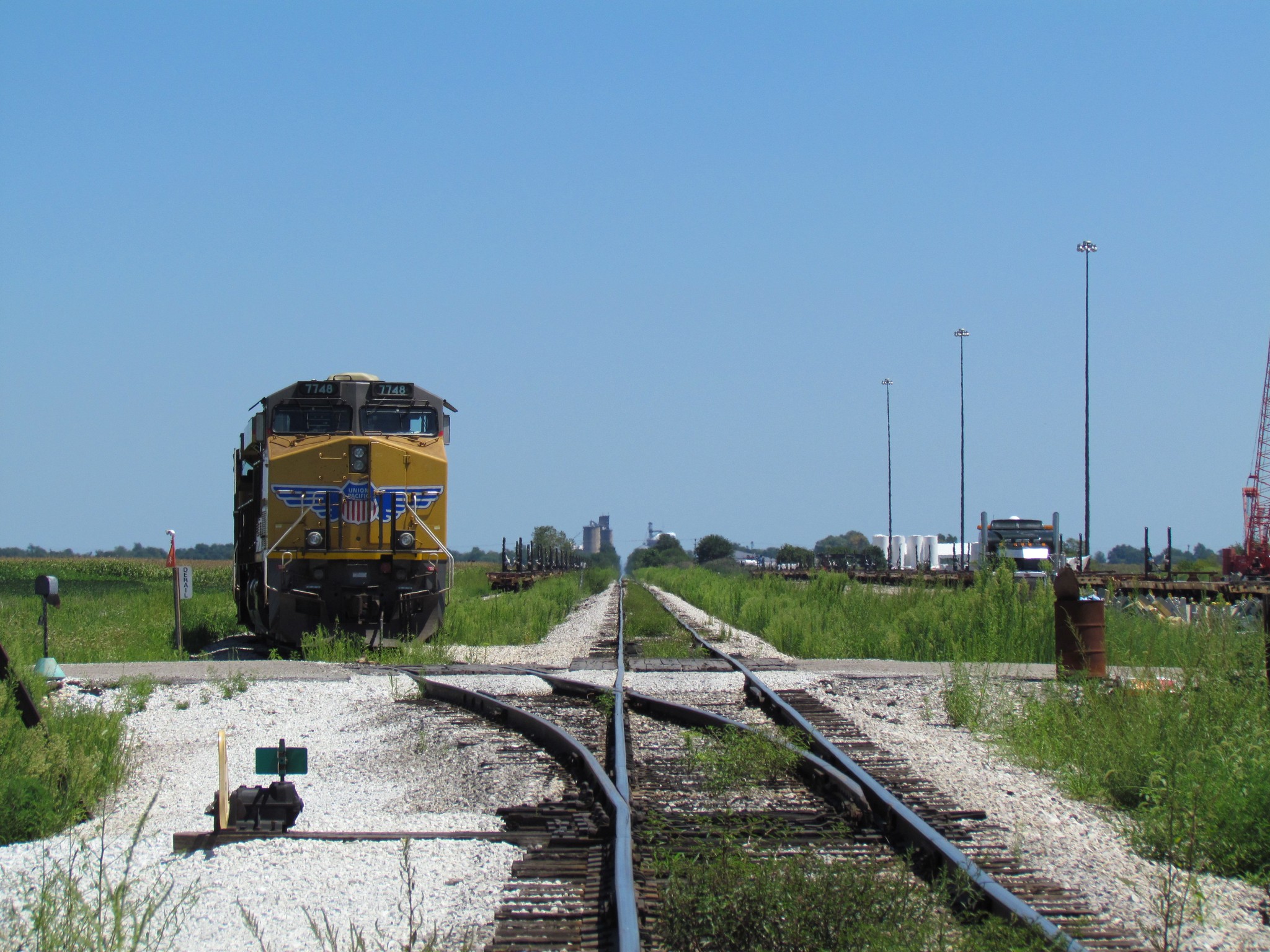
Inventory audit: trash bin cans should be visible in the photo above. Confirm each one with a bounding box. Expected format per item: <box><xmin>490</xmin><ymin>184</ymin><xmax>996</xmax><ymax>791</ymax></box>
<box><xmin>1054</xmin><ymin>567</ymin><xmax>1107</xmax><ymax>679</ymax></box>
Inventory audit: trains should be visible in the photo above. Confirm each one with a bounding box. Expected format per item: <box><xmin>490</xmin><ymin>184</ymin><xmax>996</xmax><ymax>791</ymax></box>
<box><xmin>231</xmin><ymin>372</ymin><xmax>458</xmax><ymax>652</ymax></box>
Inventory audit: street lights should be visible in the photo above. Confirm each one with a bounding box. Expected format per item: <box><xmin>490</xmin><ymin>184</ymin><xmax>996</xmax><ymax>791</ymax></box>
<box><xmin>880</xmin><ymin>378</ymin><xmax>894</xmax><ymax>571</ymax></box>
<box><xmin>954</xmin><ymin>328</ymin><xmax>969</xmax><ymax>572</ymax></box>
<box><xmin>1076</xmin><ymin>238</ymin><xmax>1098</xmax><ymax>574</ymax></box>
